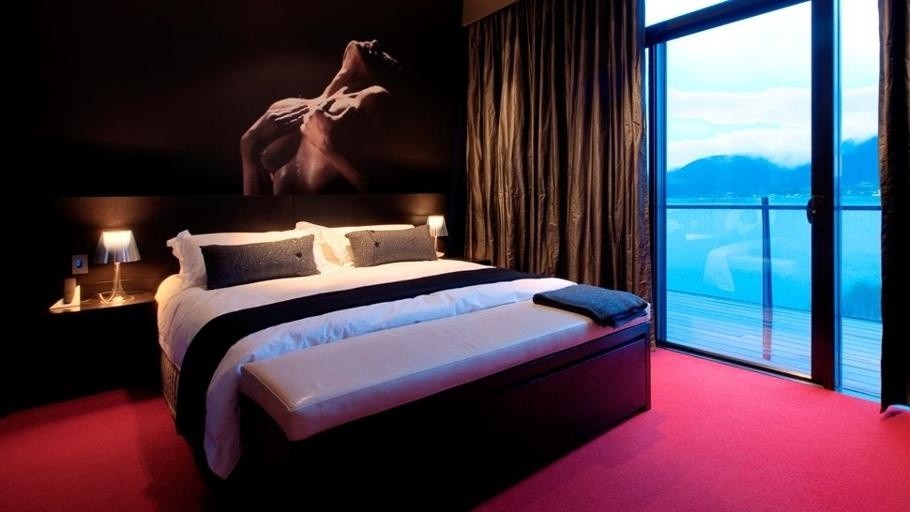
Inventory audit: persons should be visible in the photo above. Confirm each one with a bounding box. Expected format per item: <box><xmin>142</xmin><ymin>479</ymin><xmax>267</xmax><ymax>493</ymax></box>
<box><xmin>240</xmin><ymin>39</ymin><xmax>399</xmax><ymax>195</ymax></box>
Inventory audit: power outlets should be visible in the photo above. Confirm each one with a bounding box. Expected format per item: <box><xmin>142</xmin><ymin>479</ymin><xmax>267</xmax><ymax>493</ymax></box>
<box><xmin>71</xmin><ymin>254</ymin><xmax>89</xmax><ymax>276</ymax></box>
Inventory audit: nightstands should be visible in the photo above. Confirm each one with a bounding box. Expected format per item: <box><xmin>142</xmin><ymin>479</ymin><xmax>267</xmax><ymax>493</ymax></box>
<box><xmin>48</xmin><ymin>288</ymin><xmax>154</xmax><ymax>386</ymax></box>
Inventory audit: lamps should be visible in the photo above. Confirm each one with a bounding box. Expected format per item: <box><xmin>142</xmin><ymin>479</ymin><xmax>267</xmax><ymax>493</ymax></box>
<box><xmin>94</xmin><ymin>229</ymin><xmax>143</xmax><ymax>306</ymax></box>
<box><xmin>427</xmin><ymin>214</ymin><xmax>450</xmax><ymax>258</ymax></box>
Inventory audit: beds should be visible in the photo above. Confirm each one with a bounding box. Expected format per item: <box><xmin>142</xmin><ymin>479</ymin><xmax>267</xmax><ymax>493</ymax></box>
<box><xmin>152</xmin><ymin>221</ymin><xmax>656</xmax><ymax>512</ymax></box>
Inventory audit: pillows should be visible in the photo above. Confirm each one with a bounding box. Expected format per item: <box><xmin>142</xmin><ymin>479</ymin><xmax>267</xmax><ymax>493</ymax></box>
<box><xmin>164</xmin><ymin>220</ymin><xmax>320</xmax><ymax>290</ymax></box>
<box><xmin>321</xmin><ymin>223</ymin><xmax>439</xmax><ymax>269</ymax></box>
